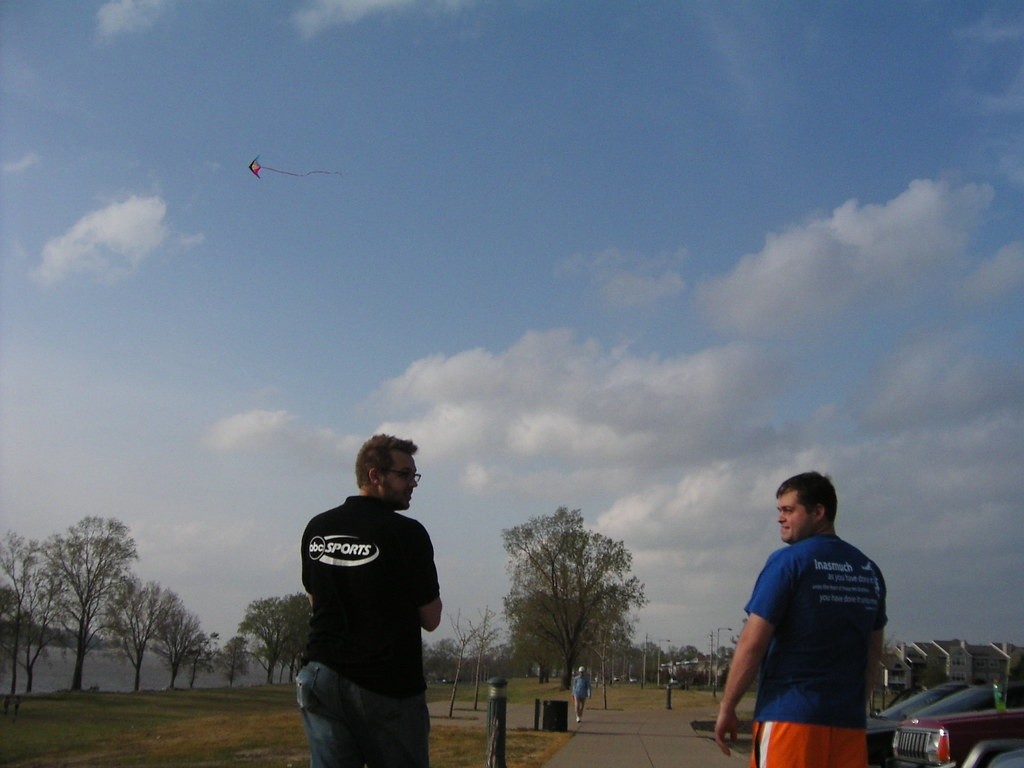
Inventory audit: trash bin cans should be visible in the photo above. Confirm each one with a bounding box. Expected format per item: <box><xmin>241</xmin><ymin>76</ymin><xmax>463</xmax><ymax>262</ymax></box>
<box><xmin>535</xmin><ymin>699</ymin><xmax>568</xmax><ymax>731</ymax></box>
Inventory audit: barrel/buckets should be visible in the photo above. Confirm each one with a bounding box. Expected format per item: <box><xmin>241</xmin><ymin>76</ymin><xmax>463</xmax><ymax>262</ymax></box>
<box><xmin>535</xmin><ymin>699</ymin><xmax>568</xmax><ymax>732</ymax></box>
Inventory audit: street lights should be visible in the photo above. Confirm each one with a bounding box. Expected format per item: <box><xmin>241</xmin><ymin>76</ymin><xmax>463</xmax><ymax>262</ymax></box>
<box><xmin>657</xmin><ymin>639</ymin><xmax>671</xmax><ymax>685</ymax></box>
<box><xmin>715</xmin><ymin>627</ymin><xmax>732</xmax><ymax>687</ymax></box>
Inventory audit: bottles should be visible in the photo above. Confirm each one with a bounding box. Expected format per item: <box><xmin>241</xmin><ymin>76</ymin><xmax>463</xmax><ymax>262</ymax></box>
<box><xmin>993</xmin><ymin>678</ymin><xmax>1006</xmax><ymax>713</ymax></box>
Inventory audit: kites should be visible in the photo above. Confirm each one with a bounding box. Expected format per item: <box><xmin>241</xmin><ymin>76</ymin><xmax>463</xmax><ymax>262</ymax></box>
<box><xmin>249</xmin><ymin>154</ymin><xmax>261</xmax><ymax>179</ymax></box>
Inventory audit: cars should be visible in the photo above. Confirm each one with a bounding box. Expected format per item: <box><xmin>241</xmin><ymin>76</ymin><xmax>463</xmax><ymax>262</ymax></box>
<box><xmin>668</xmin><ymin>679</ymin><xmax>679</xmax><ymax>685</ymax></box>
<box><xmin>867</xmin><ymin>679</ymin><xmax>1024</xmax><ymax>768</ymax></box>
<box><xmin>630</xmin><ymin>677</ymin><xmax>637</xmax><ymax>683</ymax></box>
<box><xmin>613</xmin><ymin>675</ymin><xmax>620</xmax><ymax>681</ymax></box>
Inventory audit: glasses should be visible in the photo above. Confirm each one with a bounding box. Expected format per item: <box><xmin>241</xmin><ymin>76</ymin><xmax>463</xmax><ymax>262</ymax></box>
<box><xmin>378</xmin><ymin>467</ymin><xmax>421</xmax><ymax>484</ymax></box>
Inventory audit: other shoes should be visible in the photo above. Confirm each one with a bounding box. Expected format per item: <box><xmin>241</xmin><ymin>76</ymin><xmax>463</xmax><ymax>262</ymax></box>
<box><xmin>576</xmin><ymin>717</ymin><xmax>580</xmax><ymax>723</ymax></box>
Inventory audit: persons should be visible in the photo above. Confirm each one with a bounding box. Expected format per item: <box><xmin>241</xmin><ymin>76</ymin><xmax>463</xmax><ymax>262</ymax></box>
<box><xmin>715</xmin><ymin>471</ymin><xmax>889</xmax><ymax>767</ymax></box>
<box><xmin>295</xmin><ymin>433</ymin><xmax>443</xmax><ymax>768</ymax></box>
<box><xmin>571</xmin><ymin>666</ymin><xmax>592</xmax><ymax>723</ymax></box>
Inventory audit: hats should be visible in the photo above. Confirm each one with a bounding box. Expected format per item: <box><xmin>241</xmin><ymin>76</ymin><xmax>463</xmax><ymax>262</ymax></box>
<box><xmin>579</xmin><ymin>666</ymin><xmax>585</xmax><ymax>672</ymax></box>
